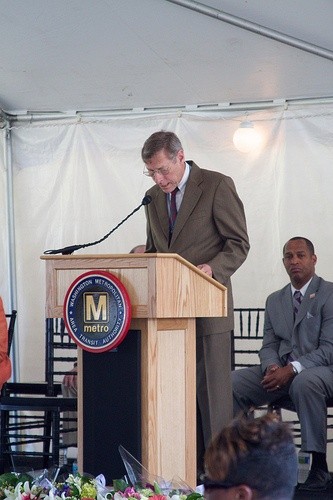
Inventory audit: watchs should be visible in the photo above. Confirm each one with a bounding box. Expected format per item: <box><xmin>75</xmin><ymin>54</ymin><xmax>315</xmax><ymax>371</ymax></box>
<box><xmin>293</xmin><ymin>367</ymin><xmax>297</xmax><ymax>374</ymax></box>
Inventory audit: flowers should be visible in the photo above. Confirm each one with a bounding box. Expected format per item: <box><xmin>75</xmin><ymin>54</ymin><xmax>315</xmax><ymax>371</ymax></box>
<box><xmin>0</xmin><ymin>469</ymin><xmax>206</xmax><ymax>500</ymax></box>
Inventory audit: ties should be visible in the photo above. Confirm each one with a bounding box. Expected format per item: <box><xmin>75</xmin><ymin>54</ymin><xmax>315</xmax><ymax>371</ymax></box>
<box><xmin>169</xmin><ymin>187</ymin><xmax>180</xmax><ymax>238</ymax></box>
<box><xmin>293</xmin><ymin>291</ymin><xmax>304</xmax><ymax>322</ymax></box>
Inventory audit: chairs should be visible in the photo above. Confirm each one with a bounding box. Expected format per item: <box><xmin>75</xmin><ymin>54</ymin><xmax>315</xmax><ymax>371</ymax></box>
<box><xmin>0</xmin><ymin>317</ymin><xmax>78</xmax><ymax>468</ymax></box>
<box><xmin>233</xmin><ymin>308</ymin><xmax>333</xmax><ymax>475</ymax></box>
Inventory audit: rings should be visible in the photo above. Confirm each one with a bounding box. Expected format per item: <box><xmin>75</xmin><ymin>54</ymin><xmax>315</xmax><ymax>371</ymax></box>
<box><xmin>276</xmin><ymin>386</ymin><xmax>280</xmax><ymax>389</ymax></box>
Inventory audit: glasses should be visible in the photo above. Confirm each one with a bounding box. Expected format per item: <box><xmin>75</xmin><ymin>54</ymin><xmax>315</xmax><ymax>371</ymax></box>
<box><xmin>143</xmin><ymin>161</ymin><xmax>172</xmax><ymax>176</ymax></box>
<box><xmin>203</xmin><ymin>476</ymin><xmax>238</xmax><ymax>490</ymax></box>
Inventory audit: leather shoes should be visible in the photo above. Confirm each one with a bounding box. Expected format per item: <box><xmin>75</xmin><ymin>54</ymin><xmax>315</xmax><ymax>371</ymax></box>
<box><xmin>298</xmin><ymin>468</ymin><xmax>332</xmax><ymax>492</ymax></box>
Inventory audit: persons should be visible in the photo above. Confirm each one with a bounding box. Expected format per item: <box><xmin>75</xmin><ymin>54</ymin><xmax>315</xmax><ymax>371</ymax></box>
<box><xmin>204</xmin><ymin>413</ymin><xmax>297</xmax><ymax>500</ymax></box>
<box><xmin>234</xmin><ymin>236</ymin><xmax>333</xmax><ymax>494</ymax></box>
<box><xmin>0</xmin><ymin>296</ymin><xmax>12</xmax><ymax>392</ymax></box>
<box><xmin>141</xmin><ymin>130</ymin><xmax>251</xmax><ymax>481</ymax></box>
<box><xmin>52</xmin><ymin>245</ymin><xmax>147</xmax><ymax>482</ymax></box>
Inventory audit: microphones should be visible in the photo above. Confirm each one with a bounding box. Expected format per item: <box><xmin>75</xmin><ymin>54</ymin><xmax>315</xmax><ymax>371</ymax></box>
<box><xmin>49</xmin><ymin>194</ymin><xmax>152</xmax><ymax>254</ymax></box>
